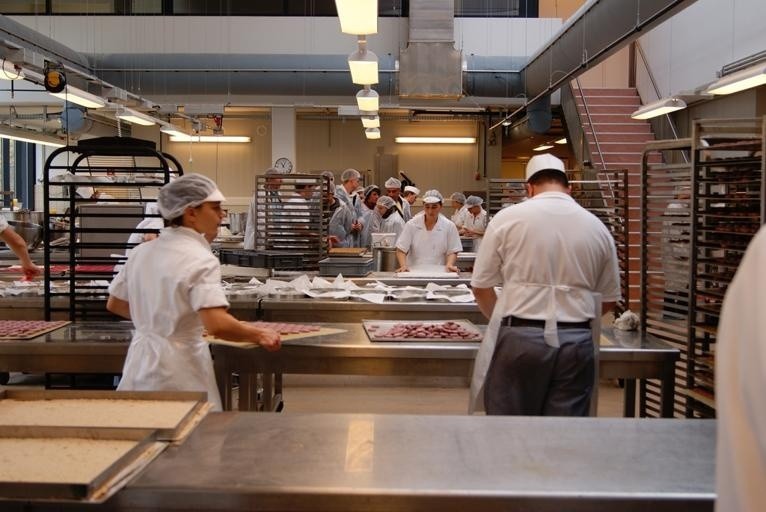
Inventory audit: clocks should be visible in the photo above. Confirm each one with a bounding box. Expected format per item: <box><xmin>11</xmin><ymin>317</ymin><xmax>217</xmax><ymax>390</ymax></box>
<box><xmin>275</xmin><ymin>158</ymin><xmax>292</xmax><ymax>174</ymax></box>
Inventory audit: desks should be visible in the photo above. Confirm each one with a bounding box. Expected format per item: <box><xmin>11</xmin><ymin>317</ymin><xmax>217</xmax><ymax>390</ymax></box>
<box><xmin>1</xmin><ymin>232</ymin><xmax>725</xmax><ymax>511</ymax></box>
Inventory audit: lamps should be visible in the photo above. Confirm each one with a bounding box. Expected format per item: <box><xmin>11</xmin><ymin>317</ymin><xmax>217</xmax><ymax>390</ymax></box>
<box><xmin>0</xmin><ymin>58</ymin><xmax>25</xmax><ymax>82</ymax></box>
<box><xmin>113</xmin><ymin>109</ymin><xmax>156</xmax><ymax>127</ymax></box>
<box><xmin>160</xmin><ymin>124</ymin><xmax>186</xmax><ymax>134</ymax></box>
<box><xmin>705</xmin><ymin>59</ymin><xmax>766</xmax><ymax>96</ymax></box>
<box><xmin>336</xmin><ymin>0</ymin><xmax>384</xmax><ymax>140</ymax></box>
<box><xmin>0</xmin><ymin>129</ymin><xmax>72</xmax><ymax>150</ymax></box>
<box><xmin>47</xmin><ymin>84</ymin><xmax>106</xmax><ymax>111</ymax></box>
<box><xmin>631</xmin><ymin>96</ymin><xmax>689</xmax><ymax>123</ymax></box>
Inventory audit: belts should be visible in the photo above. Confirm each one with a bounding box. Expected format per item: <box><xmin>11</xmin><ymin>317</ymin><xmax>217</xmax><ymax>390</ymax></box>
<box><xmin>502</xmin><ymin>316</ymin><xmax>592</xmax><ymax>329</ymax></box>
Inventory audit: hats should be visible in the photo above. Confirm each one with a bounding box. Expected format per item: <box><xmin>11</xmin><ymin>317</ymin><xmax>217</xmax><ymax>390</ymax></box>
<box><xmin>75</xmin><ymin>185</ymin><xmax>96</xmax><ymax>199</ymax></box>
<box><xmin>405</xmin><ymin>186</ymin><xmax>420</xmax><ymax>197</ymax></box>
<box><xmin>525</xmin><ymin>153</ymin><xmax>568</xmax><ymax>180</ymax></box>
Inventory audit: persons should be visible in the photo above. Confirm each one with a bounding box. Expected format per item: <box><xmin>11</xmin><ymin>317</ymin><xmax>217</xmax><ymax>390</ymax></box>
<box><xmin>104</xmin><ymin>171</ymin><xmax>282</xmax><ymax>412</ymax></box>
<box><xmin>242</xmin><ymin>166</ymin><xmax>489</xmax><ymax>274</ymax></box>
<box><xmin>713</xmin><ymin>223</ymin><xmax>766</xmax><ymax>511</ymax></box>
<box><xmin>468</xmin><ymin>153</ymin><xmax>622</xmax><ymax>417</ymax></box>
<box><xmin>112</xmin><ymin>216</ymin><xmax>165</xmax><ymax>279</ymax></box>
<box><xmin>0</xmin><ymin>213</ymin><xmax>41</xmax><ymax>278</ymax></box>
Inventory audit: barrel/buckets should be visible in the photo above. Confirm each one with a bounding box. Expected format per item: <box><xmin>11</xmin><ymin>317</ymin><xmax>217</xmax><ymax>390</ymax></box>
<box><xmin>228</xmin><ymin>211</ymin><xmax>248</xmax><ymax>234</ymax></box>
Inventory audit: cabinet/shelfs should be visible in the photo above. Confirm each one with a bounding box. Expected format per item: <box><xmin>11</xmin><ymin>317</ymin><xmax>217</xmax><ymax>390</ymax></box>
<box><xmin>254</xmin><ymin>171</ymin><xmax>328</xmax><ymax>253</ymax></box>
<box><xmin>45</xmin><ymin>135</ymin><xmax>182</xmax><ymax>389</ymax></box>
<box><xmin>486</xmin><ymin>168</ymin><xmax>630</xmax><ymax>319</ymax></box>
<box><xmin>638</xmin><ymin>119</ymin><xmax>765</xmax><ymax>418</ymax></box>
